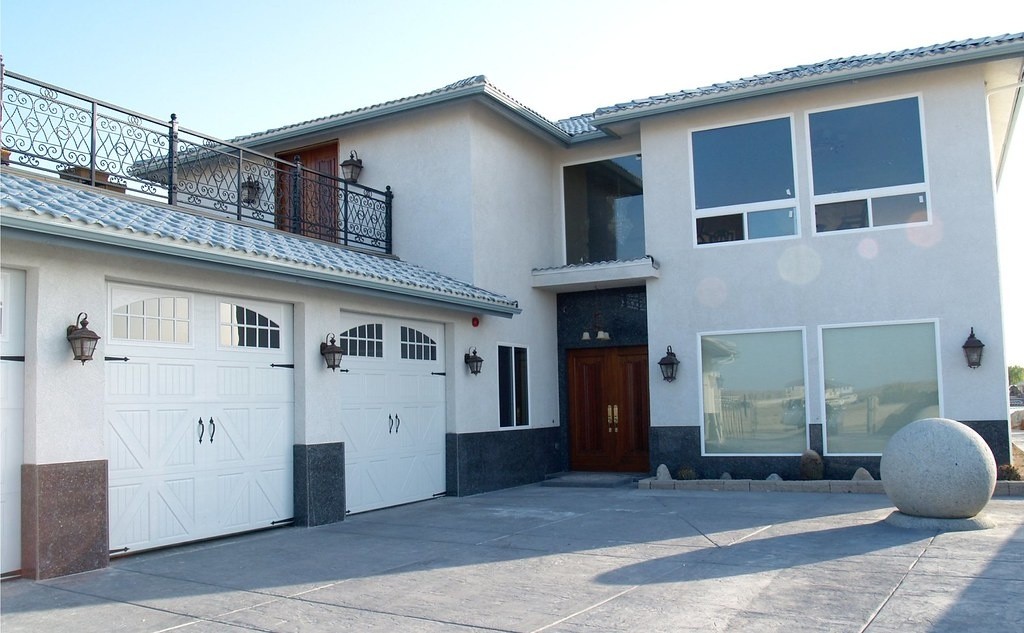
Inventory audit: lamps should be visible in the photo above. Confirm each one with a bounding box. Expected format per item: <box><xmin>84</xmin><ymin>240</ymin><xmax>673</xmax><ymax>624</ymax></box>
<box><xmin>962</xmin><ymin>327</ymin><xmax>985</xmax><ymax>370</ymax></box>
<box><xmin>320</xmin><ymin>333</ymin><xmax>343</xmax><ymax>372</ymax></box>
<box><xmin>340</xmin><ymin>149</ymin><xmax>363</xmax><ymax>183</ymax></box>
<box><xmin>658</xmin><ymin>345</ymin><xmax>680</xmax><ymax>383</ymax></box>
<box><xmin>241</xmin><ymin>176</ymin><xmax>261</xmax><ymax>205</ymax></box>
<box><xmin>581</xmin><ymin>285</ymin><xmax>612</xmax><ymax>340</ymax></box>
<box><xmin>464</xmin><ymin>346</ymin><xmax>483</xmax><ymax>376</ymax></box>
<box><xmin>67</xmin><ymin>312</ymin><xmax>102</xmax><ymax>365</ymax></box>
